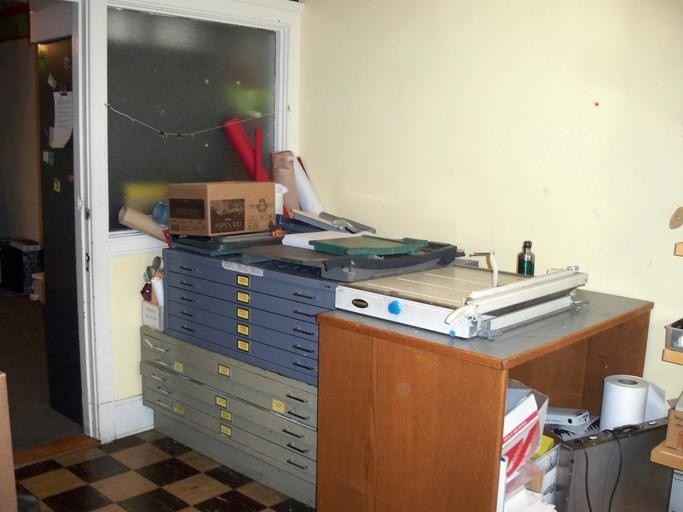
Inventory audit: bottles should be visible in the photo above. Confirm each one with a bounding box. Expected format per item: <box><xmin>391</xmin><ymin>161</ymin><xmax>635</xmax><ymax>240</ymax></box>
<box><xmin>516</xmin><ymin>240</ymin><xmax>535</xmax><ymax>276</ymax></box>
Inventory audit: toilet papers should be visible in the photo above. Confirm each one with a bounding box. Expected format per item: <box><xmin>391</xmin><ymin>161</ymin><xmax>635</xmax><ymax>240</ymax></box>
<box><xmin>292</xmin><ymin>152</ymin><xmax>323</xmax><ymax>217</ymax></box>
<box><xmin>599</xmin><ymin>374</ymin><xmax>668</xmax><ymax>434</ymax></box>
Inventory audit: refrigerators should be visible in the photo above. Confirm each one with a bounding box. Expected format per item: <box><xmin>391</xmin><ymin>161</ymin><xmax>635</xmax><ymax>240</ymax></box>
<box><xmin>33</xmin><ymin>37</ymin><xmax>84</xmax><ymax>428</ymax></box>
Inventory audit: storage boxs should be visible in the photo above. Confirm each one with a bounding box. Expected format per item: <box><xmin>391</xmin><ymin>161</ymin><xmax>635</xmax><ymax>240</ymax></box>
<box><xmin>667</xmin><ymin>399</ymin><xmax>683</xmax><ymax>457</ymax></box>
<box><xmin>664</xmin><ymin>324</ymin><xmax>683</xmax><ymax>352</ymax></box>
<box><xmin>167</xmin><ymin>180</ymin><xmax>275</xmax><ymax>238</ymax></box>
<box><xmin>501</xmin><ymin>377</ymin><xmax>549</xmax><ymax>485</ymax></box>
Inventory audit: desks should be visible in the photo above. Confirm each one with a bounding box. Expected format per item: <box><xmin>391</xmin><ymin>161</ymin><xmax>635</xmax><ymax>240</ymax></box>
<box><xmin>316</xmin><ymin>290</ymin><xmax>654</xmax><ymax>512</ymax></box>
